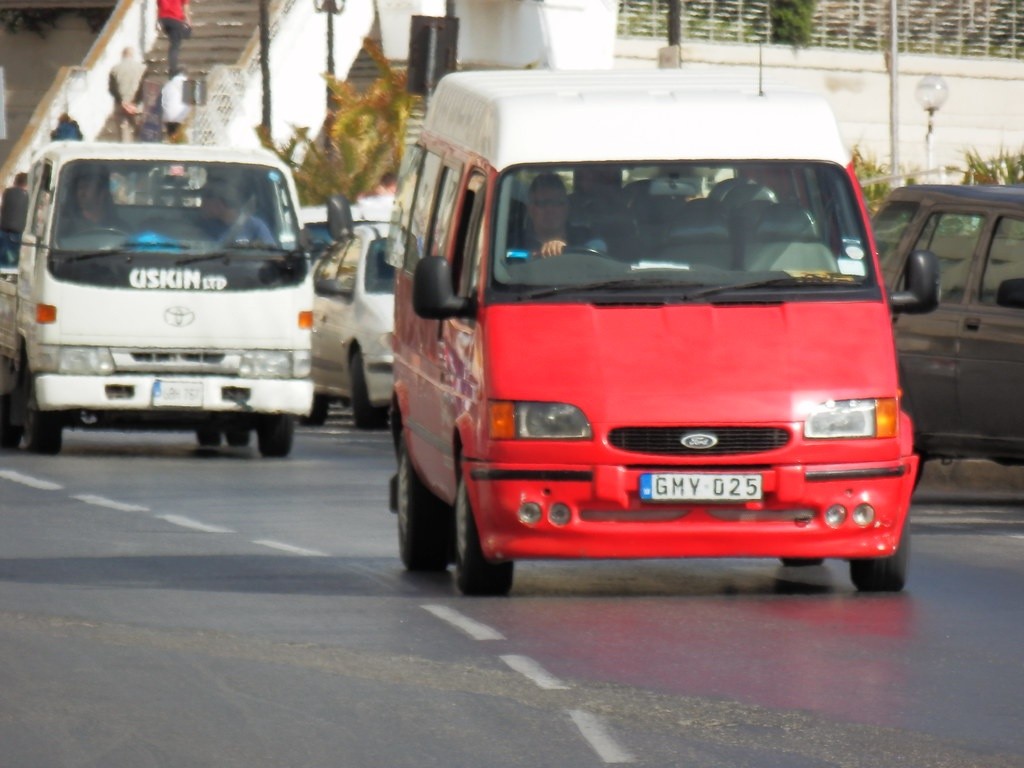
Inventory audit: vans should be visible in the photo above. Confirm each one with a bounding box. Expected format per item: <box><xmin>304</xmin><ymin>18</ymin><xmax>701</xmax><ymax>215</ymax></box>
<box><xmin>384</xmin><ymin>68</ymin><xmax>943</xmax><ymax>600</ymax></box>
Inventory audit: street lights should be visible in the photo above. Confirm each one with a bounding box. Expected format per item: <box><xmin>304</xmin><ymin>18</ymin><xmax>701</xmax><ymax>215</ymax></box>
<box><xmin>916</xmin><ymin>64</ymin><xmax>951</xmax><ymax>168</ymax></box>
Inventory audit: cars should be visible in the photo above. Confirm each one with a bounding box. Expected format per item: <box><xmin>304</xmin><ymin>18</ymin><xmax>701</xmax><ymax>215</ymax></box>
<box><xmin>866</xmin><ymin>181</ymin><xmax>1024</xmax><ymax>488</ymax></box>
<box><xmin>298</xmin><ymin>218</ymin><xmax>403</xmax><ymax>434</ymax></box>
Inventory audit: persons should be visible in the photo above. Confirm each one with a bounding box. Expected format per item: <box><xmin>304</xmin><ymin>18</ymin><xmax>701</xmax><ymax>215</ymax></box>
<box><xmin>108</xmin><ymin>46</ymin><xmax>145</xmax><ymax>143</ymax></box>
<box><xmin>513</xmin><ymin>171</ymin><xmax>607</xmax><ymax>261</ymax></box>
<box><xmin>196</xmin><ymin>178</ymin><xmax>275</xmax><ymax>249</ymax></box>
<box><xmin>156</xmin><ymin>0</ymin><xmax>193</xmax><ymax>75</ymax></box>
<box><xmin>0</xmin><ymin>172</ymin><xmax>30</xmax><ymax>268</ymax></box>
<box><xmin>49</xmin><ymin>116</ymin><xmax>82</xmax><ymax>141</ymax></box>
<box><xmin>160</xmin><ymin>68</ymin><xmax>192</xmax><ymax>136</ymax></box>
<box><xmin>63</xmin><ymin>163</ymin><xmax>132</xmax><ymax>238</ymax></box>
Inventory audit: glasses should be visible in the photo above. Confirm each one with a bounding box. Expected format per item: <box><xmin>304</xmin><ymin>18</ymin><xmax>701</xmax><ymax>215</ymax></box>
<box><xmin>529</xmin><ymin>196</ymin><xmax>567</xmax><ymax>208</ymax></box>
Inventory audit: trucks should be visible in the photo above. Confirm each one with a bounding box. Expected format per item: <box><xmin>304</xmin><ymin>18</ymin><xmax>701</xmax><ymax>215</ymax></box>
<box><xmin>0</xmin><ymin>138</ymin><xmax>352</xmax><ymax>461</ymax></box>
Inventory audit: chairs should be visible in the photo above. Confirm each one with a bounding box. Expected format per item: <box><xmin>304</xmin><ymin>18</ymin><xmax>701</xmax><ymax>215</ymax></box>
<box><xmin>602</xmin><ymin>177</ymin><xmax>836</xmax><ymax>274</ymax></box>
